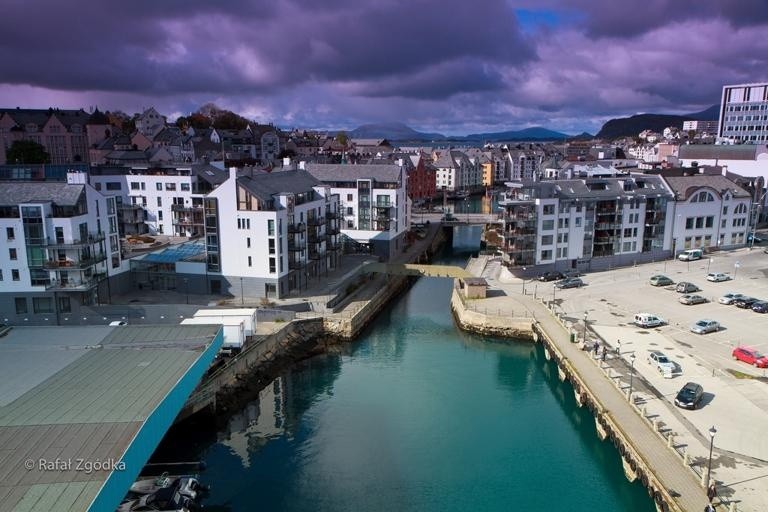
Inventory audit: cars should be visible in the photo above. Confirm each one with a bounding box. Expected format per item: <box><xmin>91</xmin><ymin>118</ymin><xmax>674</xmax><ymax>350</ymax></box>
<box><xmin>440</xmin><ymin>213</ymin><xmax>458</xmax><ymax>222</ymax></box>
<box><xmin>747</xmin><ymin>231</ymin><xmax>762</xmax><ymax>243</ymax></box>
<box><xmin>649</xmin><ymin>271</ymin><xmax>768</xmax><ymax>314</ymax></box>
<box><xmin>730</xmin><ymin>345</ymin><xmax>768</xmax><ymax>369</ymax></box>
<box><xmin>552</xmin><ymin>277</ymin><xmax>583</xmax><ymax>289</ymax></box>
<box><xmin>108</xmin><ymin>320</ymin><xmax>127</xmax><ymax>326</ymax></box>
<box><xmin>689</xmin><ymin>317</ymin><xmax>721</xmax><ymax>335</ymax></box>
<box><xmin>561</xmin><ymin>269</ymin><xmax>581</xmax><ymax>279</ymax></box>
<box><xmin>537</xmin><ymin>270</ymin><xmax>564</xmax><ymax>282</ymax></box>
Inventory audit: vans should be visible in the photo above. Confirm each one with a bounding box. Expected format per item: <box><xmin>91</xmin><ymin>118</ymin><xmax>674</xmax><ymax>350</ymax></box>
<box><xmin>678</xmin><ymin>248</ymin><xmax>704</xmax><ymax>262</ymax></box>
<box><xmin>633</xmin><ymin>312</ymin><xmax>665</xmax><ymax>329</ymax></box>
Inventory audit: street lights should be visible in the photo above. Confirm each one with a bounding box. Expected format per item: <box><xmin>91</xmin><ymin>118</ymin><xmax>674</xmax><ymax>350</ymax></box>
<box><xmin>552</xmin><ymin>281</ymin><xmax>558</xmax><ymax>307</ymax></box>
<box><xmin>467</xmin><ymin>202</ymin><xmax>470</xmax><ymax>224</ymax></box>
<box><xmin>708</xmin><ymin>424</ymin><xmax>719</xmax><ymax>479</ymax></box>
<box><xmin>630</xmin><ymin>350</ymin><xmax>636</xmax><ymax>395</ymax></box>
<box><xmin>582</xmin><ymin>310</ymin><xmax>588</xmax><ymax>342</ymax></box>
<box><xmin>184</xmin><ymin>278</ymin><xmax>189</xmax><ymax>304</ymax></box>
<box><xmin>463</xmin><ymin>197</ymin><xmax>467</xmax><ymax>213</ymax></box>
<box><xmin>522</xmin><ymin>266</ymin><xmax>527</xmax><ymax>295</ymax></box>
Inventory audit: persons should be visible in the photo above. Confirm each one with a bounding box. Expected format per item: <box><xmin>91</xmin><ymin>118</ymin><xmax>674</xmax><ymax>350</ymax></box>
<box><xmin>593</xmin><ymin>340</ymin><xmax>599</xmax><ymax>356</ymax></box>
<box><xmin>601</xmin><ymin>346</ymin><xmax>608</xmax><ymax>362</ymax></box>
<box><xmin>706</xmin><ymin>484</ymin><xmax>717</xmax><ymax>503</ymax></box>
<box><xmin>704</xmin><ymin>501</ymin><xmax>718</xmax><ymax>512</ymax></box>
<box><xmin>615</xmin><ymin>339</ymin><xmax>621</xmax><ymax>356</ymax></box>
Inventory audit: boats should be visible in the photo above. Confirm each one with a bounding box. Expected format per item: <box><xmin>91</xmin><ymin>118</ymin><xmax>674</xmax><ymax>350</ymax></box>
<box><xmin>487</xmin><ymin>190</ymin><xmax>498</xmax><ymax>196</ymax></box>
<box><xmin>456</xmin><ymin>189</ymin><xmax>470</xmax><ymax>199</ymax></box>
<box><xmin>114</xmin><ymin>473</ymin><xmax>210</xmax><ymax>512</ymax></box>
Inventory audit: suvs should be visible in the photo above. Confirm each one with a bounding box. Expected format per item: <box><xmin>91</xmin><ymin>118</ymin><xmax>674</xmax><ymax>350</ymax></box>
<box><xmin>673</xmin><ymin>381</ymin><xmax>704</xmax><ymax>410</ymax></box>
<box><xmin>647</xmin><ymin>350</ymin><xmax>677</xmax><ymax>375</ymax></box>
